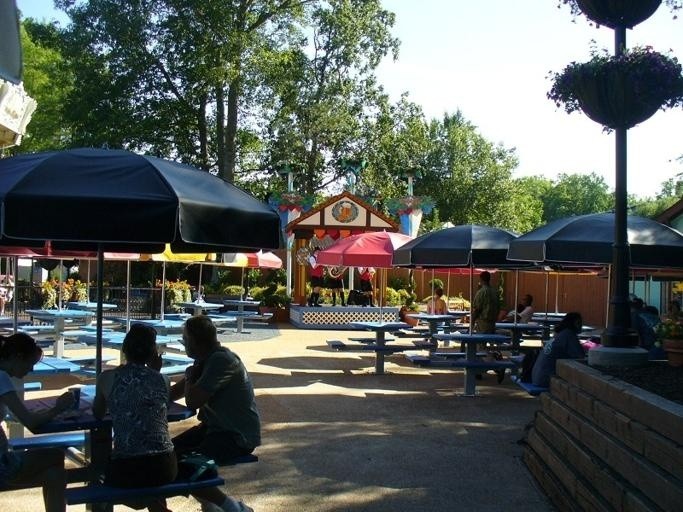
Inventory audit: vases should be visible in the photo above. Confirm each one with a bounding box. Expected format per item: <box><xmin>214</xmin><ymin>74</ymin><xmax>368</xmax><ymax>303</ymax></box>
<box><xmin>662</xmin><ymin>340</ymin><xmax>683</xmax><ymax>365</ymax></box>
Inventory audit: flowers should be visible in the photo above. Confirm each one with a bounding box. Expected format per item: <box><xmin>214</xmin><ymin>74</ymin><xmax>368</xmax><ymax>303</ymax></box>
<box><xmin>652</xmin><ymin>283</ymin><xmax>683</xmax><ymax>349</ymax></box>
<box><xmin>547</xmin><ymin>45</ymin><xmax>683</xmax><ymax>134</ymax></box>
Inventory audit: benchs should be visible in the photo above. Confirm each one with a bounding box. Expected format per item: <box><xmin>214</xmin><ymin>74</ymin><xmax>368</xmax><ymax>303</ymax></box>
<box><xmin>263</xmin><ymin>313</ymin><xmax>273</xmax><ymax>319</ymax></box>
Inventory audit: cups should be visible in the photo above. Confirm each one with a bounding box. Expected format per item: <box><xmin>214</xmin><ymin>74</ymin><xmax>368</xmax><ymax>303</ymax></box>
<box><xmin>68</xmin><ymin>388</ymin><xmax>80</xmax><ymax>409</ymax></box>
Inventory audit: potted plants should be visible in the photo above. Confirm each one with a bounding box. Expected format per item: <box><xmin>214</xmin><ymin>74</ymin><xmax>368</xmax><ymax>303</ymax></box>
<box><xmin>400</xmin><ymin>293</ymin><xmax>420</xmax><ymax>327</ymax></box>
<box><xmin>259</xmin><ymin>282</ymin><xmax>292</xmax><ymax>323</ymax></box>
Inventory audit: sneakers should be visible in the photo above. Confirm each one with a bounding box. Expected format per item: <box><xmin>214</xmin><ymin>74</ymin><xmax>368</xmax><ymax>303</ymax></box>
<box><xmin>239</xmin><ymin>501</ymin><xmax>254</xmax><ymax>512</ymax></box>
<box><xmin>332</xmin><ymin>302</ymin><xmax>346</xmax><ymax>306</ymax></box>
<box><xmin>363</xmin><ymin>303</ymin><xmax>376</xmax><ymax>307</ymax></box>
<box><xmin>308</xmin><ymin>303</ymin><xmax>322</xmax><ymax>307</ymax></box>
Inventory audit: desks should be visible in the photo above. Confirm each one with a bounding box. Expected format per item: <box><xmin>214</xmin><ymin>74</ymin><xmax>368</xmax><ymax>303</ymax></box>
<box><xmin>349</xmin><ymin>312</ymin><xmax>604</xmax><ymax>398</ymax></box>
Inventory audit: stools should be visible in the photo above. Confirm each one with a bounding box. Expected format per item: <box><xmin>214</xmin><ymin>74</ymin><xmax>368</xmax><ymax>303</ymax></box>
<box><xmin>326</xmin><ymin>341</ymin><xmax>346</xmax><ymax>351</ymax></box>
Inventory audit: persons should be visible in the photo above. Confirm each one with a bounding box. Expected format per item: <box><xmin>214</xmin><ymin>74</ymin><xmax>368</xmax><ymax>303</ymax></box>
<box><xmin>307</xmin><ymin>246</ymin><xmax>325</xmax><ymax>307</ymax></box>
<box><xmin>530</xmin><ymin>311</ymin><xmax>586</xmax><ymax>387</ymax></box>
<box><xmin>0</xmin><ymin>333</ymin><xmax>79</xmax><ymax>511</ymax></box>
<box><xmin>90</xmin><ymin>323</ymin><xmax>182</xmax><ymax>512</ymax></box>
<box><xmin>166</xmin><ymin>316</ymin><xmax>260</xmax><ymax>511</ymax></box>
<box><xmin>329</xmin><ymin>266</ymin><xmax>349</xmax><ymax>307</ymax></box>
<box><xmin>358</xmin><ymin>266</ymin><xmax>377</xmax><ymax>308</ymax></box>
<box><xmin>469</xmin><ymin>271</ymin><xmax>500</xmax><ymax>351</ymax></box>
<box><xmin>629</xmin><ymin>297</ymin><xmax>667</xmax><ymax>361</ymax></box>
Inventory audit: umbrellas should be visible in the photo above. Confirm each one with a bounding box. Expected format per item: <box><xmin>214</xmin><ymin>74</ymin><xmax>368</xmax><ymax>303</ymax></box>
<box><xmin>387</xmin><ymin>209</ymin><xmax>683</xmax><ymax>331</ymax></box>
<box><xmin>313</xmin><ymin>230</ymin><xmax>418</xmax><ymax>324</ymax></box>
